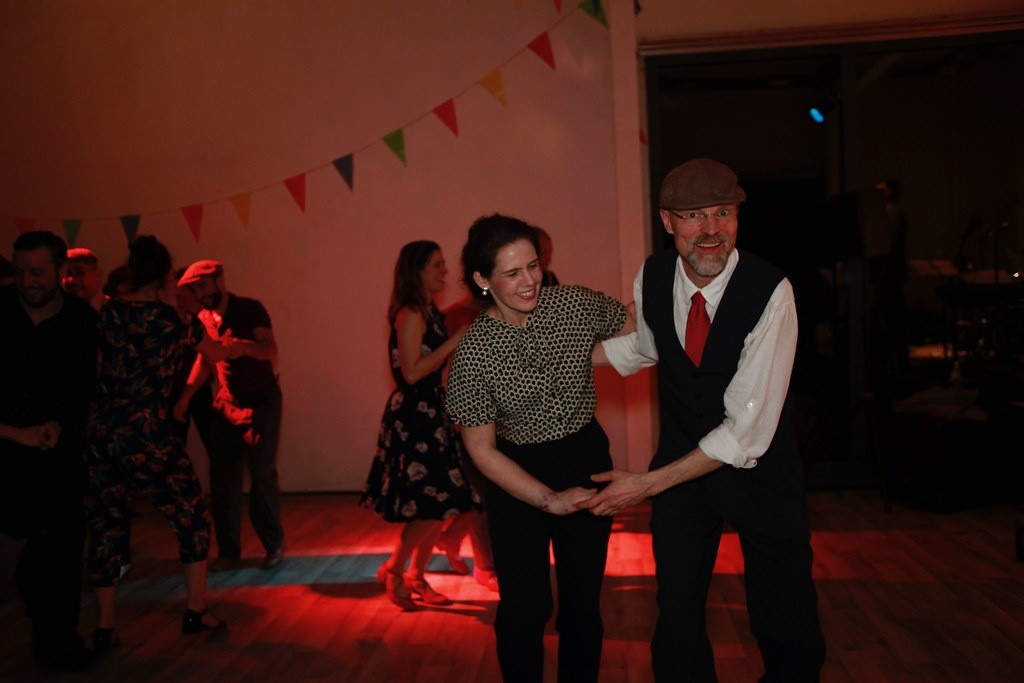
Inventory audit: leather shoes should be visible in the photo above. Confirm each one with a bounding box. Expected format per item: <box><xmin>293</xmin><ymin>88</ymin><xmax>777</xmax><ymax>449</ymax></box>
<box><xmin>93</xmin><ymin>626</ymin><xmax>123</xmax><ymax>654</ymax></box>
<box><xmin>182</xmin><ymin>608</ymin><xmax>228</xmax><ymax>633</ymax></box>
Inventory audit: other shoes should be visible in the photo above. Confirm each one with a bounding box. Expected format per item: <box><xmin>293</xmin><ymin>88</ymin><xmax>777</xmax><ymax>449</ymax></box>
<box><xmin>212</xmin><ymin>556</ymin><xmax>232</xmax><ymax>572</ymax></box>
<box><xmin>263</xmin><ymin>549</ymin><xmax>283</xmax><ymax>570</ymax></box>
<box><xmin>33</xmin><ymin>632</ymin><xmax>98</xmax><ymax>671</ymax></box>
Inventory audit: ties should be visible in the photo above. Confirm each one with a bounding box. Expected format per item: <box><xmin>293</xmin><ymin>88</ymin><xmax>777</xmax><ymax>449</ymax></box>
<box><xmin>685</xmin><ymin>291</ymin><xmax>712</xmax><ymax>368</ymax></box>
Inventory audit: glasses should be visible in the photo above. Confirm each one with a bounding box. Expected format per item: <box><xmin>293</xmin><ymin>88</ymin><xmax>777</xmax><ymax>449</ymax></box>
<box><xmin>667</xmin><ymin>207</ymin><xmax>740</xmax><ymax>222</ymax></box>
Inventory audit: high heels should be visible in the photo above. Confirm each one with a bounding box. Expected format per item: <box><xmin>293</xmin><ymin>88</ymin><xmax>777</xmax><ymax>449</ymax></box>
<box><xmin>435</xmin><ymin>532</ymin><xmax>471</xmax><ymax>575</ymax></box>
<box><xmin>376</xmin><ymin>561</ymin><xmax>454</xmax><ymax>611</ymax></box>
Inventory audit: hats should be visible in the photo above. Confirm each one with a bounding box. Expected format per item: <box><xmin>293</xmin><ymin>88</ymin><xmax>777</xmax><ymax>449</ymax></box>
<box><xmin>175</xmin><ymin>259</ymin><xmax>224</xmax><ymax>288</ymax></box>
<box><xmin>658</xmin><ymin>157</ymin><xmax>747</xmax><ymax>211</ymax></box>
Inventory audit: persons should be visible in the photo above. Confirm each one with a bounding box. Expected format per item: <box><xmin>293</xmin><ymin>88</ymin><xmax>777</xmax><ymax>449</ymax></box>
<box><xmin>61</xmin><ymin>247</ymin><xmax>107</xmax><ymax>311</ymax></box>
<box><xmin>0</xmin><ymin>231</ymin><xmax>101</xmax><ymax>675</ymax></box>
<box><xmin>571</xmin><ymin>157</ymin><xmax>826</xmax><ymax>683</ymax></box>
<box><xmin>366</xmin><ymin>241</ymin><xmax>467</xmax><ymax>612</ymax></box>
<box><xmin>87</xmin><ymin>235</ymin><xmax>226</xmax><ymax>652</ymax></box>
<box><xmin>445</xmin><ymin>215</ymin><xmax>637</xmax><ymax>683</ymax></box>
<box><xmin>173</xmin><ymin>260</ymin><xmax>282</xmax><ymax>574</ymax></box>
